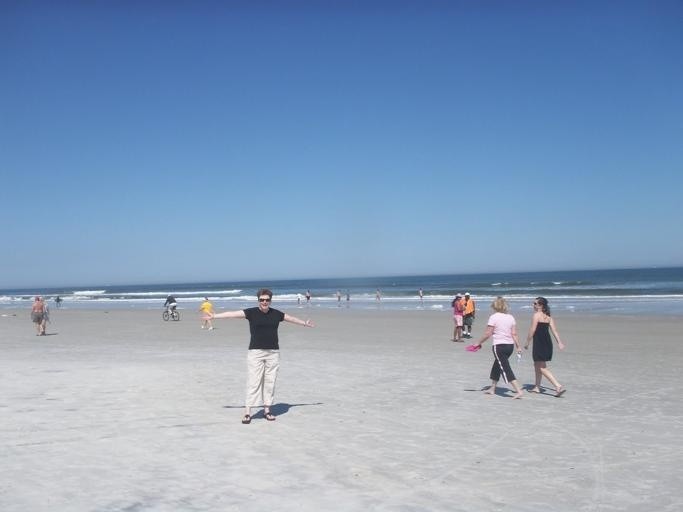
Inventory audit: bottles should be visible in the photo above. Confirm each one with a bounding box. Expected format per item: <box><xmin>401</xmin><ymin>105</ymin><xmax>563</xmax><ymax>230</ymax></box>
<box><xmin>516</xmin><ymin>352</ymin><xmax>522</xmax><ymax>362</ymax></box>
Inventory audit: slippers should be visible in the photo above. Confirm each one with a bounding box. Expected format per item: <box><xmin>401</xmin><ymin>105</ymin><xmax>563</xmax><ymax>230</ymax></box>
<box><xmin>263</xmin><ymin>413</ymin><xmax>275</xmax><ymax>421</ymax></box>
<box><xmin>242</xmin><ymin>415</ymin><xmax>250</xmax><ymax>424</ymax></box>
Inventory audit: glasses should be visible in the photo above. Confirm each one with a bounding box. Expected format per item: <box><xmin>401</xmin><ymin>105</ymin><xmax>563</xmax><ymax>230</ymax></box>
<box><xmin>259</xmin><ymin>299</ymin><xmax>270</xmax><ymax>302</ymax></box>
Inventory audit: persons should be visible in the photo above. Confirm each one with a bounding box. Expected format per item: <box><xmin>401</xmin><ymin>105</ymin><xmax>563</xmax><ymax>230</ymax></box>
<box><xmin>375</xmin><ymin>289</ymin><xmax>380</xmax><ymax>301</ymax></box>
<box><xmin>30</xmin><ymin>297</ymin><xmax>45</xmax><ymax>335</ymax></box>
<box><xmin>295</xmin><ymin>292</ymin><xmax>301</xmax><ymax>303</ymax></box>
<box><xmin>199</xmin><ymin>289</ymin><xmax>315</xmax><ymax>423</ymax></box>
<box><xmin>305</xmin><ymin>289</ymin><xmax>312</xmax><ymax>303</ymax></box>
<box><xmin>418</xmin><ymin>287</ymin><xmax>423</xmax><ymax>299</ymax></box>
<box><xmin>39</xmin><ymin>299</ymin><xmax>51</xmax><ymax>332</ymax></box>
<box><xmin>524</xmin><ymin>297</ymin><xmax>567</xmax><ymax>397</ymax></box>
<box><xmin>460</xmin><ymin>292</ymin><xmax>476</xmax><ymax>338</ymax></box>
<box><xmin>451</xmin><ymin>293</ymin><xmax>466</xmax><ymax>342</ymax></box>
<box><xmin>163</xmin><ymin>294</ymin><xmax>176</xmax><ymax>317</ymax></box>
<box><xmin>473</xmin><ymin>297</ymin><xmax>523</xmax><ymax>400</ymax></box>
<box><xmin>195</xmin><ymin>297</ymin><xmax>214</xmax><ymax>330</ymax></box>
<box><xmin>337</xmin><ymin>289</ymin><xmax>341</xmax><ymax>303</ymax></box>
<box><xmin>345</xmin><ymin>290</ymin><xmax>350</xmax><ymax>300</ymax></box>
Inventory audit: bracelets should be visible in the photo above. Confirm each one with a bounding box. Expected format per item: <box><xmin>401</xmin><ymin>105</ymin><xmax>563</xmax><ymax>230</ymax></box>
<box><xmin>303</xmin><ymin>320</ymin><xmax>306</xmax><ymax>327</ymax></box>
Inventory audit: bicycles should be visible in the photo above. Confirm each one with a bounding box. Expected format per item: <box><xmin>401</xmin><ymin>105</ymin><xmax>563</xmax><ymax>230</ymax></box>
<box><xmin>162</xmin><ymin>305</ymin><xmax>179</xmax><ymax>321</ymax></box>
